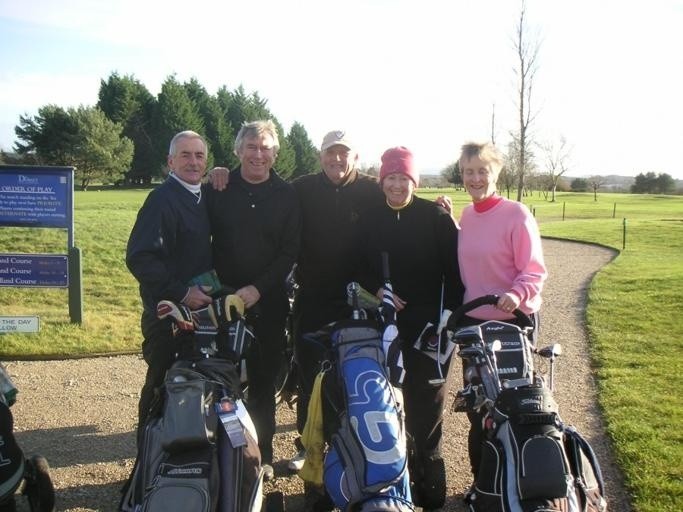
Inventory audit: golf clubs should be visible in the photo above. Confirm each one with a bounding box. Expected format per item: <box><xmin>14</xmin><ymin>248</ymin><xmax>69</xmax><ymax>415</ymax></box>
<box><xmin>427</xmin><ymin>270</ymin><xmax>447</xmax><ymax>388</ymax></box>
<box><xmin>453</xmin><ymin>325</ymin><xmax>508</xmax><ymax>413</ymax></box>
<box><xmin>549</xmin><ymin>343</ymin><xmax>562</xmax><ymax>396</ymax></box>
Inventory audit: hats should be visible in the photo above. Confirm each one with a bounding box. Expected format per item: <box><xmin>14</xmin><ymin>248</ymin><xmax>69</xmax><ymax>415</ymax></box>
<box><xmin>380</xmin><ymin>147</ymin><xmax>420</xmax><ymax>188</ymax></box>
<box><xmin>322</xmin><ymin>130</ymin><xmax>354</xmax><ymax>151</ymax></box>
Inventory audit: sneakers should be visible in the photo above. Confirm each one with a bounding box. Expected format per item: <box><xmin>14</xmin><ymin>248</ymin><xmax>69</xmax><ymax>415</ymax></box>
<box><xmin>287</xmin><ymin>449</ymin><xmax>307</xmax><ymax>470</ymax></box>
<box><xmin>262</xmin><ymin>463</ymin><xmax>274</xmax><ymax>482</ymax></box>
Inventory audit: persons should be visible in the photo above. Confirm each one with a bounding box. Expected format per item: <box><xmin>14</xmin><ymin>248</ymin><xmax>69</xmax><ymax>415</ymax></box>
<box><xmin>347</xmin><ymin>145</ymin><xmax>463</xmax><ymax>511</ymax></box>
<box><xmin>200</xmin><ymin>117</ymin><xmax>304</xmax><ymax>483</ymax></box>
<box><xmin>122</xmin><ymin>129</ymin><xmax>229</xmax><ymax>458</ymax></box>
<box><xmin>286</xmin><ymin>128</ymin><xmax>384</xmax><ymax>471</ymax></box>
<box><xmin>433</xmin><ymin>141</ymin><xmax>548</xmax><ymax>503</ymax></box>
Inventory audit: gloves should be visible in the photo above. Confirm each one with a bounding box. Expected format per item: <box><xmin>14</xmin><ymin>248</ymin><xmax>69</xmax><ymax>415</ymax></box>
<box><xmin>435</xmin><ymin>309</ymin><xmax>454</xmax><ymax>341</ymax></box>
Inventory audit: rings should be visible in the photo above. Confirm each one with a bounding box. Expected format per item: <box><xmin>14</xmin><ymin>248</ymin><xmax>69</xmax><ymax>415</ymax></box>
<box><xmin>243</xmin><ymin>302</ymin><xmax>246</xmax><ymax>304</ymax></box>
<box><xmin>506</xmin><ymin>306</ymin><xmax>511</xmax><ymax>310</ymax></box>
